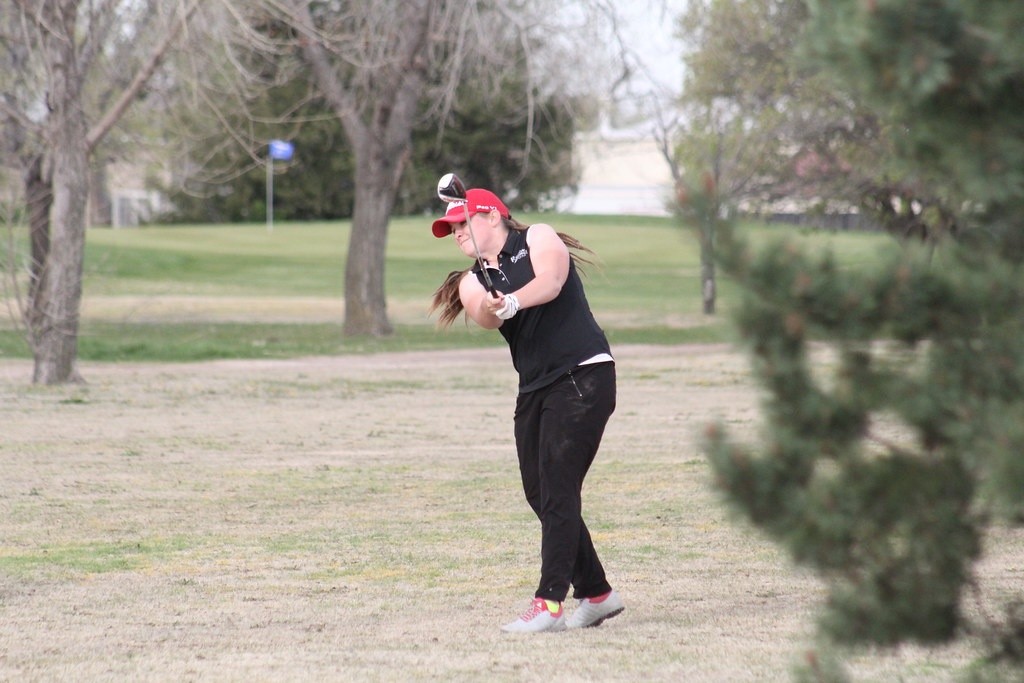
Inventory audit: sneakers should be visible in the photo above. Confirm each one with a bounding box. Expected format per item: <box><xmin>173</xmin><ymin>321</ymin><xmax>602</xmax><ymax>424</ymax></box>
<box><xmin>500</xmin><ymin>595</ymin><xmax>567</xmax><ymax>636</ymax></box>
<box><xmin>566</xmin><ymin>590</ymin><xmax>626</xmax><ymax>631</ymax></box>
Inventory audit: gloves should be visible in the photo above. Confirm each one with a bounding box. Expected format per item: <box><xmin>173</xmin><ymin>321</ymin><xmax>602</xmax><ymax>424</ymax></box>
<box><xmin>496</xmin><ymin>294</ymin><xmax>520</xmax><ymax>320</ymax></box>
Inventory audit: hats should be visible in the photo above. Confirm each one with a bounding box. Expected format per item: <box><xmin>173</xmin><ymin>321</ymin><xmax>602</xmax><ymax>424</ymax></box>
<box><xmin>432</xmin><ymin>187</ymin><xmax>510</xmax><ymax>238</ymax></box>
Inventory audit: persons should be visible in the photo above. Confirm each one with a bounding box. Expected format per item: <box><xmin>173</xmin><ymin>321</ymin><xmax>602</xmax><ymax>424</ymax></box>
<box><xmin>430</xmin><ymin>188</ymin><xmax>625</xmax><ymax>632</ymax></box>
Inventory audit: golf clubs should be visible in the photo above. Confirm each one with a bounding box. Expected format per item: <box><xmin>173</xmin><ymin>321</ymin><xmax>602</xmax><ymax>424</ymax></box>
<box><xmin>437</xmin><ymin>171</ymin><xmax>499</xmax><ymax>299</ymax></box>
